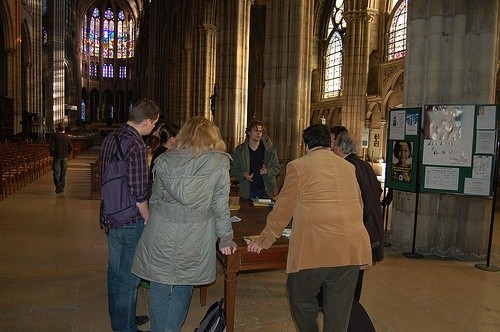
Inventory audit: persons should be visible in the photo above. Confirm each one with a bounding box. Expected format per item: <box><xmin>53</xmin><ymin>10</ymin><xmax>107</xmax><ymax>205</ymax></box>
<box><xmin>229</xmin><ymin>119</ymin><xmax>282</xmax><ymax>197</ymax></box>
<box><xmin>100</xmin><ymin>98</ymin><xmax>160</xmax><ymax>332</ymax></box>
<box><xmin>145</xmin><ymin>119</ymin><xmax>179</xmax><ymax>201</ymax></box>
<box><xmin>249</xmin><ymin>123</ymin><xmax>374</xmax><ymax>332</ymax></box>
<box><xmin>50</xmin><ymin>123</ymin><xmax>72</xmax><ymax>194</ymax></box>
<box><xmin>331</xmin><ymin>132</ymin><xmax>385</xmax><ymax>332</ymax></box>
<box><xmin>130</xmin><ymin>116</ymin><xmax>239</xmax><ymax>332</ymax></box>
<box><xmin>315</xmin><ymin>125</ymin><xmax>348</xmax><ymax>314</ymax></box>
<box><xmin>394</xmin><ymin>141</ymin><xmax>412</xmax><ymax>165</ymax></box>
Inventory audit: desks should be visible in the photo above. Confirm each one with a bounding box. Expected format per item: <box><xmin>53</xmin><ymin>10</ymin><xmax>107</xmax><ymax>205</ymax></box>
<box><xmin>68</xmin><ymin>133</ymin><xmax>98</xmax><ymax>156</ymax></box>
<box><xmin>96</xmin><ymin>127</ymin><xmax>118</xmax><ymax>137</ymax></box>
<box><xmin>200</xmin><ymin>199</ymin><xmax>391</xmax><ymax>332</ymax></box>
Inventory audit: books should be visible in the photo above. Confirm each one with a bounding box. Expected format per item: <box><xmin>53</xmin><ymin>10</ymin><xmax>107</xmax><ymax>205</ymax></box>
<box><xmin>251</xmin><ymin>194</ymin><xmax>275</xmax><ymax>207</ymax></box>
<box><xmin>229</xmin><ymin>195</ymin><xmax>240</xmax><ymax>211</ymax></box>
<box><xmin>243</xmin><ymin>235</ymin><xmax>261</xmax><ymax>243</ymax></box>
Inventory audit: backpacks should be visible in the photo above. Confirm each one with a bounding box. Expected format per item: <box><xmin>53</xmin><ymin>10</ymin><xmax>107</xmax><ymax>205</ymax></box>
<box><xmin>99</xmin><ymin>132</ymin><xmax>141</xmax><ymax>235</ymax></box>
<box><xmin>193</xmin><ymin>297</ymin><xmax>226</xmax><ymax>332</ymax></box>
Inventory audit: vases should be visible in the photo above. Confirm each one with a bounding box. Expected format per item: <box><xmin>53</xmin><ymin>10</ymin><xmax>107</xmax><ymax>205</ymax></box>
<box><xmin>105</xmin><ymin>114</ymin><xmax>113</xmax><ymax>127</ymax></box>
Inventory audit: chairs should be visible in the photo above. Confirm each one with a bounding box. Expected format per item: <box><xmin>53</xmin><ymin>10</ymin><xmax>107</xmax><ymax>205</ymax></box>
<box><xmin>89</xmin><ymin>152</ymin><xmax>239</xmax><ymax>199</ymax></box>
<box><xmin>0</xmin><ymin>141</ymin><xmax>79</xmax><ymax>201</ymax></box>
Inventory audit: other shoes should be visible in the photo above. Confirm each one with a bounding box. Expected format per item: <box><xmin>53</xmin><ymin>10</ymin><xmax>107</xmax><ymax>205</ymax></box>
<box><xmin>55</xmin><ymin>182</ymin><xmax>64</xmax><ymax>194</ymax></box>
<box><xmin>136</xmin><ymin>314</ymin><xmax>149</xmax><ymax>326</ymax></box>
